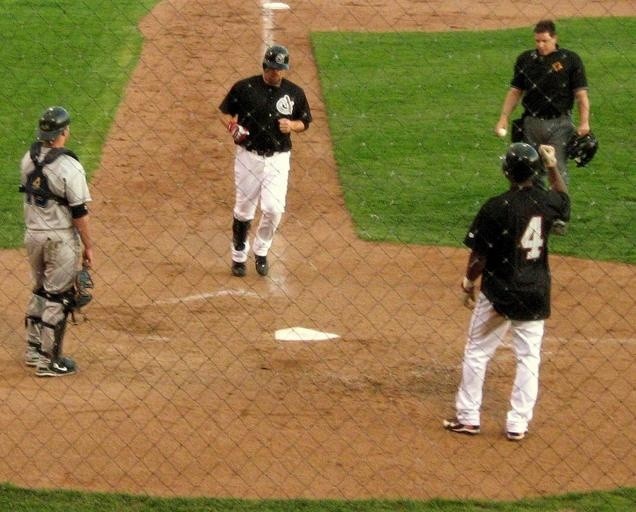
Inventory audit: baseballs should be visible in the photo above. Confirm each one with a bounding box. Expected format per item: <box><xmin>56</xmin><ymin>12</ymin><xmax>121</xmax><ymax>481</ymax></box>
<box><xmin>498</xmin><ymin>128</ymin><xmax>506</xmax><ymax>137</ymax></box>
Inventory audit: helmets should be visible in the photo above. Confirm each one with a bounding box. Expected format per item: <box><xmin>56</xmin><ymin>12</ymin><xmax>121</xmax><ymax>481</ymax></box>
<box><xmin>261</xmin><ymin>45</ymin><xmax>294</xmax><ymax>72</ymax></box>
<box><xmin>35</xmin><ymin>106</ymin><xmax>75</xmax><ymax>139</ymax></box>
<box><xmin>567</xmin><ymin>130</ymin><xmax>602</xmax><ymax>167</ymax></box>
<box><xmin>502</xmin><ymin>141</ymin><xmax>542</xmax><ymax>182</ymax></box>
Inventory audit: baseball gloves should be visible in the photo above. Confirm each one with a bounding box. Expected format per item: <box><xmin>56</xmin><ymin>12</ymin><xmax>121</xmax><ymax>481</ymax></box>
<box><xmin>565</xmin><ymin>132</ymin><xmax>598</xmax><ymax>168</ymax></box>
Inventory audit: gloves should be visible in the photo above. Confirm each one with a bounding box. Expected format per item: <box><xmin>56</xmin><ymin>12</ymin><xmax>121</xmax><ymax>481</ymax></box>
<box><xmin>537</xmin><ymin>144</ymin><xmax>558</xmax><ymax>169</ymax></box>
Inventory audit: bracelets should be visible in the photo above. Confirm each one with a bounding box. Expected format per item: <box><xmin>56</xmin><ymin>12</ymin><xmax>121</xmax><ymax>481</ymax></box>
<box><xmin>459</xmin><ymin>281</ymin><xmax>474</xmax><ymax>292</ymax></box>
<box><xmin>501</xmin><ymin>110</ymin><xmax>509</xmax><ymax>117</ymax></box>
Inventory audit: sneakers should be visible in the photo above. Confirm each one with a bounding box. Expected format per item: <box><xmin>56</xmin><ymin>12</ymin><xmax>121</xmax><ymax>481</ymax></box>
<box><xmin>32</xmin><ymin>356</ymin><xmax>77</xmax><ymax>380</ymax></box>
<box><xmin>20</xmin><ymin>338</ymin><xmax>61</xmax><ymax>369</ymax></box>
<box><xmin>502</xmin><ymin>423</ymin><xmax>536</xmax><ymax>445</ymax></box>
<box><xmin>230</xmin><ymin>259</ymin><xmax>252</xmax><ymax>276</ymax></box>
<box><xmin>441</xmin><ymin>415</ymin><xmax>490</xmax><ymax>444</ymax></box>
<box><xmin>253</xmin><ymin>257</ymin><xmax>272</xmax><ymax>276</ymax></box>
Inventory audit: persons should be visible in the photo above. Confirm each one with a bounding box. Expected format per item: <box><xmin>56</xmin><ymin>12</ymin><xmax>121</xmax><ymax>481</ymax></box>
<box><xmin>18</xmin><ymin>106</ymin><xmax>92</xmax><ymax>375</ymax></box>
<box><xmin>495</xmin><ymin>19</ymin><xmax>591</xmax><ymax>230</ymax></box>
<box><xmin>218</xmin><ymin>45</ymin><xmax>313</xmax><ymax>276</ymax></box>
<box><xmin>442</xmin><ymin>141</ymin><xmax>571</xmax><ymax>440</ymax></box>
